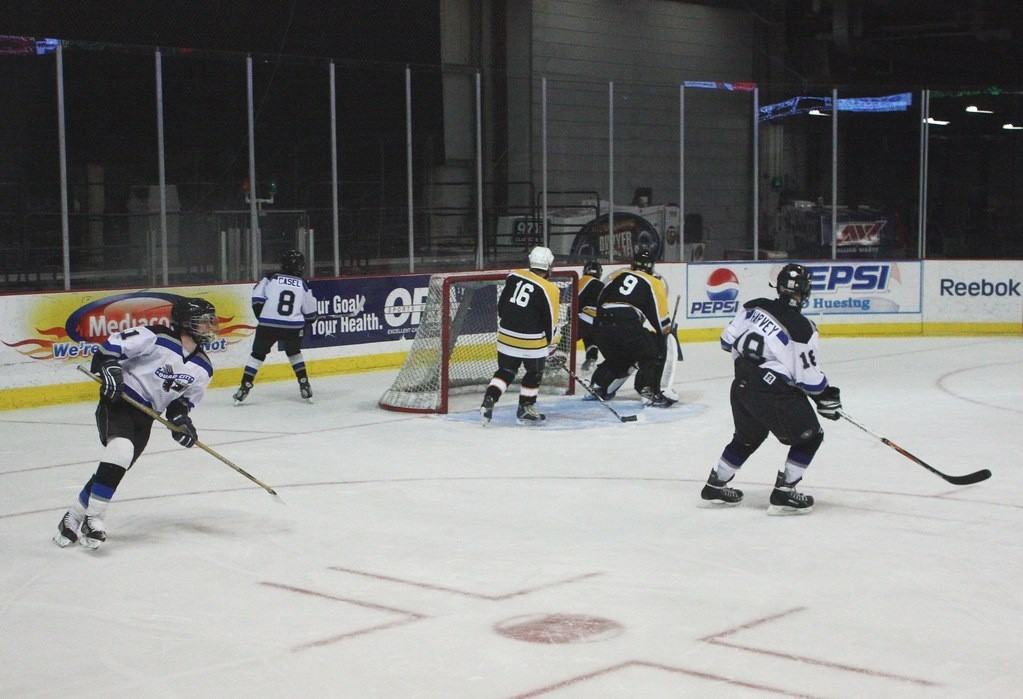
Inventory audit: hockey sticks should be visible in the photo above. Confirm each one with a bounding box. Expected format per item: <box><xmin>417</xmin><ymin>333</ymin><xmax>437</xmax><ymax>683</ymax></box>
<box><xmin>836</xmin><ymin>408</ymin><xmax>992</xmax><ymax>485</ymax></box>
<box><xmin>552</xmin><ymin>355</ymin><xmax>645</xmax><ymax>423</ymax></box>
<box><xmin>75</xmin><ymin>364</ymin><xmax>289</xmax><ymax>520</ymax></box>
<box><xmin>670</xmin><ymin>294</ymin><xmax>682</xmax><ymax>333</ymax></box>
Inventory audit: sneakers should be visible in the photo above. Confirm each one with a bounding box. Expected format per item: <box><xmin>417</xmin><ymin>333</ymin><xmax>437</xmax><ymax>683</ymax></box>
<box><xmin>699</xmin><ymin>467</ymin><xmax>744</xmax><ymax>508</ymax></box>
<box><xmin>641</xmin><ymin>386</ymin><xmax>673</xmax><ymax>408</ymax></box>
<box><xmin>232</xmin><ymin>380</ymin><xmax>254</xmax><ymax>406</ymax></box>
<box><xmin>515</xmin><ymin>402</ymin><xmax>547</xmax><ymax>425</ymax></box>
<box><xmin>767</xmin><ymin>470</ymin><xmax>815</xmax><ymax>516</ymax></box>
<box><xmin>78</xmin><ymin>514</ymin><xmax>106</xmax><ymax>552</ymax></box>
<box><xmin>581</xmin><ymin>359</ymin><xmax>598</xmax><ymax>374</ymax></box>
<box><xmin>300</xmin><ymin>376</ymin><xmax>313</xmax><ymax>403</ymax></box>
<box><xmin>480</xmin><ymin>393</ymin><xmax>495</xmax><ymax>427</ymax></box>
<box><xmin>583</xmin><ymin>387</ymin><xmax>617</xmax><ymax>401</ymax></box>
<box><xmin>53</xmin><ymin>512</ymin><xmax>82</xmax><ymax>548</ymax></box>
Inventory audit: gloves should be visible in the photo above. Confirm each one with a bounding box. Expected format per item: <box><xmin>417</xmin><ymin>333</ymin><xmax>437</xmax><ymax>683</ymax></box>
<box><xmin>167</xmin><ymin>406</ymin><xmax>198</xmax><ymax>448</ymax></box>
<box><xmin>100</xmin><ymin>360</ymin><xmax>123</xmax><ymax>403</ymax></box>
<box><xmin>816</xmin><ymin>385</ymin><xmax>842</xmax><ymax>421</ymax></box>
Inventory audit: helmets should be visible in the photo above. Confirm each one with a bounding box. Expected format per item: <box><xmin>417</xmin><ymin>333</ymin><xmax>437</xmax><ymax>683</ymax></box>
<box><xmin>528</xmin><ymin>245</ymin><xmax>554</xmax><ymax>272</ymax></box>
<box><xmin>583</xmin><ymin>262</ymin><xmax>603</xmax><ymax>279</ymax></box>
<box><xmin>630</xmin><ymin>250</ymin><xmax>654</xmax><ymax>276</ymax></box>
<box><xmin>281</xmin><ymin>250</ymin><xmax>305</xmax><ymax>276</ymax></box>
<box><xmin>776</xmin><ymin>263</ymin><xmax>811</xmax><ymax>307</ymax></box>
<box><xmin>168</xmin><ymin>297</ymin><xmax>220</xmax><ymax>345</ymax></box>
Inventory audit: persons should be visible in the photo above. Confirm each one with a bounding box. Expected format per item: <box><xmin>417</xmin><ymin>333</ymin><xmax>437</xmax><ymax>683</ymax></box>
<box><xmin>478</xmin><ymin>246</ymin><xmax>561</xmax><ymax>425</ymax></box>
<box><xmin>584</xmin><ymin>251</ymin><xmax>680</xmax><ymax>412</ymax></box>
<box><xmin>549</xmin><ymin>259</ymin><xmax>607</xmax><ymax>372</ymax></box>
<box><xmin>695</xmin><ymin>264</ymin><xmax>844</xmax><ymax>517</ymax></box>
<box><xmin>51</xmin><ymin>298</ymin><xmax>216</xmax><ymax>550</ymax></box>
<box><xmin>231</xmin><ymin>248</ymin><xmax>318</xmax><ymax>405</ymax></box>
